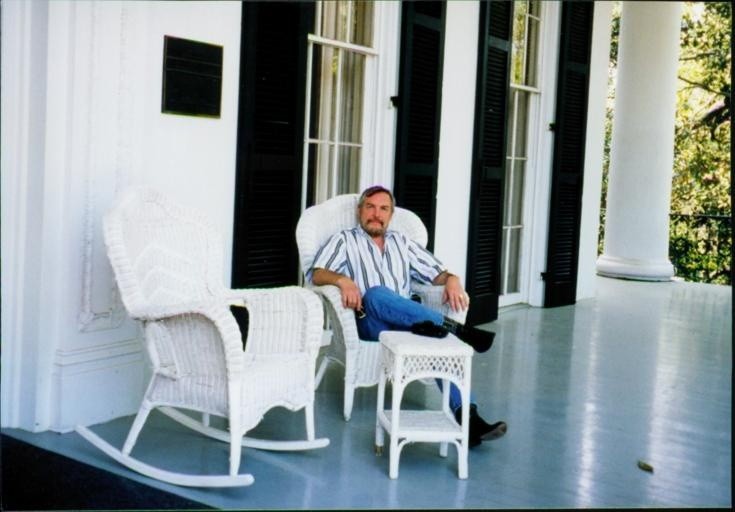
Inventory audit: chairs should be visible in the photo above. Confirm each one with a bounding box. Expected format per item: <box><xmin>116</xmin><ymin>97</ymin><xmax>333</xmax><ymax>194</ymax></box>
<box><xmin>296</xmin><ymin>194</ymin><xmax>472</xmax><ymax>422</ymax></box>
<box><xmin>74</xmin><ymin>195</ymin><xmax>330</xmax><ymax>488</ymax></box>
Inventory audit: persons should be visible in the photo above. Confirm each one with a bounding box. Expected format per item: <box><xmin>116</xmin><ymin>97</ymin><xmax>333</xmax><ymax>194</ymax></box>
<box><xmin>303</xmin><ymin>185</ymin><xmax>508</xmax><ymax>450</ymax></box>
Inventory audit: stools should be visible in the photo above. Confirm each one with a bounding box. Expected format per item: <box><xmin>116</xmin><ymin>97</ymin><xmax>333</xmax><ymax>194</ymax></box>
<box><xmin>375</xmin><ymin>326</ymin><xmax>475</xmax><ymax>480</ymax></box>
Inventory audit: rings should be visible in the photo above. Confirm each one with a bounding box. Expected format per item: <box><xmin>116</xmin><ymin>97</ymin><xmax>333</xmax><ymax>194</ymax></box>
<box><xmin>459</xmin><ymin>294</ymin><xmax>463</xmax><ymax>297</ymax></box>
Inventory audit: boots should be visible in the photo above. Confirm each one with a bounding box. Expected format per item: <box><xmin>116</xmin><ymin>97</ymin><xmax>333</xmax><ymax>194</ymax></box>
<box><xmin>455</xmin><ymin>323</ymin><xmax>496</xmax><ymax>353</ymax></box>
<box><xmin>455</xmin><ymin>404</ymin><xmax>507</xmax><ymax>449</ymax></box>
